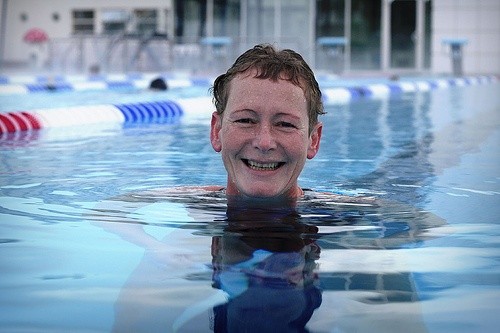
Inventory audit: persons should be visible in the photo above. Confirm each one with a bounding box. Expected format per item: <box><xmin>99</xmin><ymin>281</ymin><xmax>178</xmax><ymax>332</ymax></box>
<box><xmin>84</xmin><ymin>185</ymin><xmax>452</xmax><ymax>333</ymax></box>
<box><xmin>149</xmin><ymin>78</ymin><xmax>168</xmax><ymax>91</ymax></box>
<box><xmin>206</xmin><ymin>45</ymin><xmax>328</xmax><ymax>198</ymax></box>
